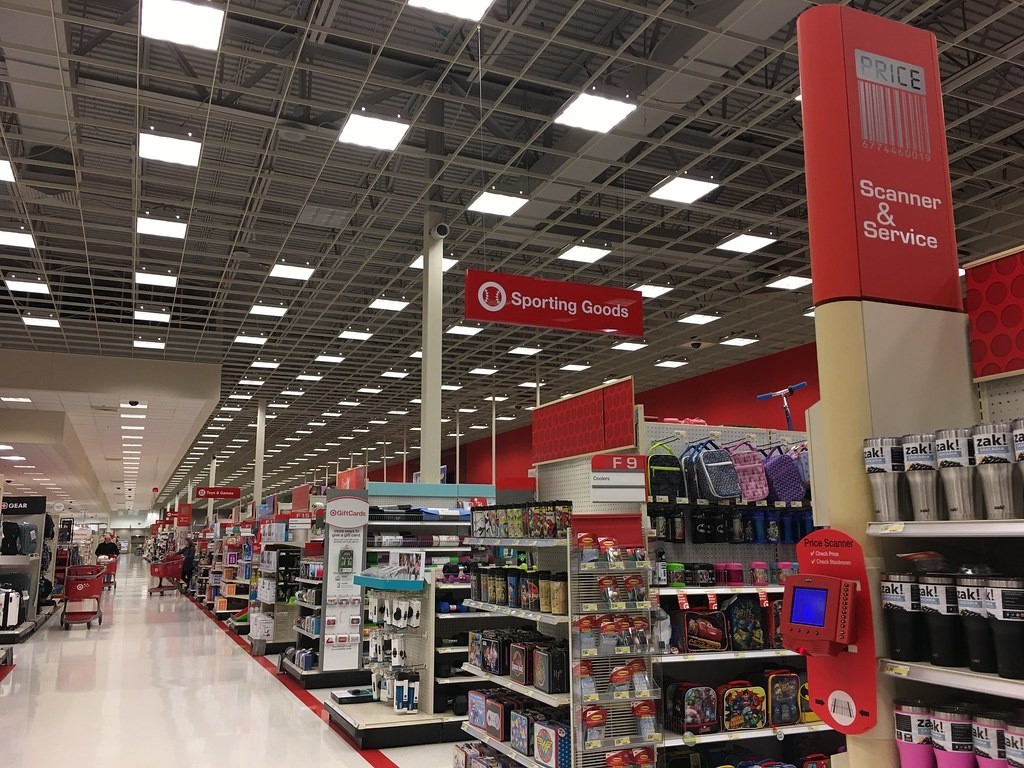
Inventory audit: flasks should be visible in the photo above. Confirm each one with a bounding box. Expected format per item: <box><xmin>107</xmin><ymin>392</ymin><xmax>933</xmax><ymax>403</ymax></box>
<box><xmin>465</xmin><ymin>499</ymin><xmax>572</xmax><ymax>617</ymax></box>
<box><xmin>861</xmin><ymin>415</ymin><xmax>1024</xmax><ymax>768</ymax></box>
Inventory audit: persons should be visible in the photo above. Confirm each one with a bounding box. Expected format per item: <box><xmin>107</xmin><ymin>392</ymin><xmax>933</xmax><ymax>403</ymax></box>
<box><xmin>94</xmin><ymin>533</ymin><xmax>120</xmax><ymax>591</ymax></box>
<box><xmin>173</xmin><ymin>538</ymin><xmax>197</xmax><ymax>591</ymax></box>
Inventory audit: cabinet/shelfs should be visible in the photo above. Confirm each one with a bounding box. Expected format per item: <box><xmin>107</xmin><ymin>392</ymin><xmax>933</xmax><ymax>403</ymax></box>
<box><xmin>1</xmin><ymin>494</ymin><xmax>109</xmax><ymax>682</ymax></box>
<box><xmin>142</xmin><ymin>375</ymin><xmax>847</xmax><ymax>768</ymax></box>
<box><xmin>866</xmin><ymin>518</ymin><xmax>1024</xmax><ymax>705</ymax></box>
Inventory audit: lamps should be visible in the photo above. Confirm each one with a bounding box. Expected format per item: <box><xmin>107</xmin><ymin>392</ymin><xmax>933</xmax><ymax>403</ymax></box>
<box><xmin>1</xmin><ymin>3</ymin><xmax>815</xmax><ymax>534</ymax></box>
<box><xmin>958</xmin><ymin>267</ymin><xmax>965</xmax><ymax>278</ymax></box>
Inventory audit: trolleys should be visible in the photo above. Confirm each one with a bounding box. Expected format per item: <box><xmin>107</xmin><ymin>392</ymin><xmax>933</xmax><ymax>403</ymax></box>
<box><xmin>96</xmin><ymin>553</ymin><xmax>119</xmax><ymax>591</ymax></box>
<box><xmin>147</xmin><ymin>553</ymin><xmax>185</xmax><ymax>597</ymax></box>
<box><xmin>60</xmin><ymin>564</ymin><xmax>107</xmax><ymax>629</ymax></box>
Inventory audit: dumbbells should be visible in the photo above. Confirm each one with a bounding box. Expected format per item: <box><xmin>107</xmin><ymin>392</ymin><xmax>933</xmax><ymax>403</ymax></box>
<box><xmin>432</xmin><ymin>571</ymin><xmax>475</xmax><ymax>716</ymax></box>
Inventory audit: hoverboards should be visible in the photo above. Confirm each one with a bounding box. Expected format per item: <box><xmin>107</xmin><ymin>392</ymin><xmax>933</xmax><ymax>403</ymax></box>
<box><xmin>756</xmin><ymin>382</ymin><xmax>808</xmax><ymax>431</ymax></box>
